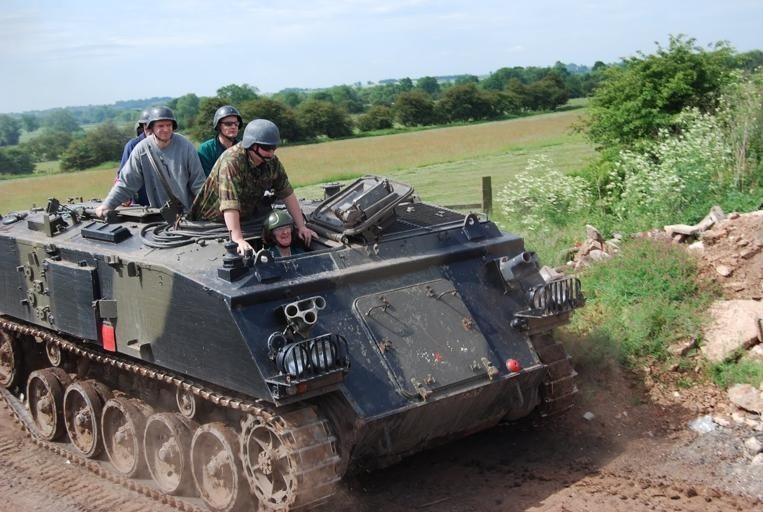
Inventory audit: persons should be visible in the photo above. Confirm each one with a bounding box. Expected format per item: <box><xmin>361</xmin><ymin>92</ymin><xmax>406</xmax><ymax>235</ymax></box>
<box><xmin>198</xmin><ymin>105</ymin><xmax>243</xmax><ymax>181</ymax></box>
<box><xmin>263</xmin><ymin>209</ymin><xmax>305</xmax><ymax>258</ymax></box>
<box><xmin>116</xmin><ymin>110</ymin><xmax>150</xmax><ymax>206</ymax></box>
<box><xmin>95</xmin><ymin>106</ymin><xmax>206</xmax><ymax>219</ymax></box>
<box><xmin>190</xmin><ymin>119</ymin><xmax>320</xmax><ymax>258</ymax></box>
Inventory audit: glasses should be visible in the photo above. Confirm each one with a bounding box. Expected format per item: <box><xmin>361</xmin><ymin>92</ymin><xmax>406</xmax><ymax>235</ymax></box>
<box><xmin>220</xmin><ymin>120</ymin><xmax>240</xmax><ymax>126</ymax></box>
<box><xmin>258</xmin><ymin>145</ymin><xmax>277</xmax><ymax>152</ymax></box>
<box><xmin>274</xmin><ymin>224</ymin><xmax>294</xmax><ymax>232</ymax></box>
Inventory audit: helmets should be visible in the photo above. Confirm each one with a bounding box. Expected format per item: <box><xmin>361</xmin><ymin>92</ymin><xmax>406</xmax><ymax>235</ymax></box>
<box><xmin>146</xmin><ymin>106</ymin><xmax>177</xmax><ymax>130</ymax></box>
<box><xmin>138</xmin><ymin>108</ymin><xmax>149</xmax><ymax>126</ymax></box>
<box><xmin>241</xmin><ymin>119</ymin><xmax>280</xmax><ymax>149</ymax></box>
<box><xmin>213</xmin><ymin>106</ymin><xmax>242</xmax><ymax>131</ymax></box>
<box><xmin>263</xmin><ymin>209</ymin><xmax>295</xmax><ymax>232</ymax></box>
